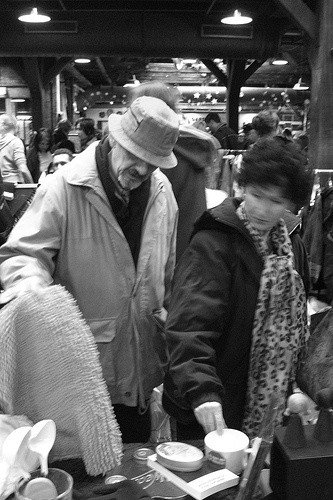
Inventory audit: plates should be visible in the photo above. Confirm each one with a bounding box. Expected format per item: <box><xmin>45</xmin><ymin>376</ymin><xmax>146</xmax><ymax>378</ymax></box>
<box><xmin>156</xmin><ymin>442</ymin><xmax>203</xmax><ymax>472</ymax></box>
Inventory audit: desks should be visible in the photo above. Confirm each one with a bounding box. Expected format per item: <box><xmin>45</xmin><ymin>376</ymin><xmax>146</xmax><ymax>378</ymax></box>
<box><xmin>47</xmin><ymin>441</ymin><xmax>242</xmax><ymax>500</ymax></box>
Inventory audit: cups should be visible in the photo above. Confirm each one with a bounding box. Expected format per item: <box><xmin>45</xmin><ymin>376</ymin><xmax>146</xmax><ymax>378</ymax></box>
<box><xmin>14</xmin><ymin>467</ymin><xmax>74</xmax><ymax>500</ymax></box>
<box><xmin>203</xmin><ymin>428</ymin><xmax>253</xmax><ymax>475</ymax></box>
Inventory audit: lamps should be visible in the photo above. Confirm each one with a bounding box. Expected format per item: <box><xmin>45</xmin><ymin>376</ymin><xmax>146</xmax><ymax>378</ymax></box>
<box><xmin>221</xmin><ymin>9</ymin><xmax>252</xmax><ymax>25</ymax></box>
<box><xmin>18</xmin><ymin>8</ymin><xmax>51</xmax><ymax>22</ymax></box>
<box><xmin>293</xmin><ymin>46</ymin><xmax>310</xmax><ymax>90</ymax></box>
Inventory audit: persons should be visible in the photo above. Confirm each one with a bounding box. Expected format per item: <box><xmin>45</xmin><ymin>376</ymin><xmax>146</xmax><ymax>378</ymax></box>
<box><xmin>0</xmin><ymin>114</ymin><xmax>35</xmax><ymax>185</ymax></box>
<box><xmin>204</xmin><ymin>112</ymin><xmax>240</xmax><ymax>149</ymax></box>
<box><xmin>132</xmin><ymin>79</ymin><xmax>221</xmax><ymax>266</ymax></box>
<box><xmin>1</xmin><ymin>96</ymin><xmax>180</xmax><ymax>441</ymax></box>
<box><xmin>27</xmin><ymin>118</ymin><xmax>99</xmax><ymax>184</ymax></box>
<box><xmin>161</xmin><ymin>137</ymin><xmax>315</xmax><ymax>445</ymax></box>
<box><xmin>240</xmin><ymin>109</ymin><xmax>310</xmax><ymax>165</ymax></box>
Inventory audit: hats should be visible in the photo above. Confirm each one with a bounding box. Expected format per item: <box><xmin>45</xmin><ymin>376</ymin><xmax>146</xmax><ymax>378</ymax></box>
<box><xmin>107</xmin><ymin>95</ymin><xmax>181</xmax><ymax>168</ymax></box>
